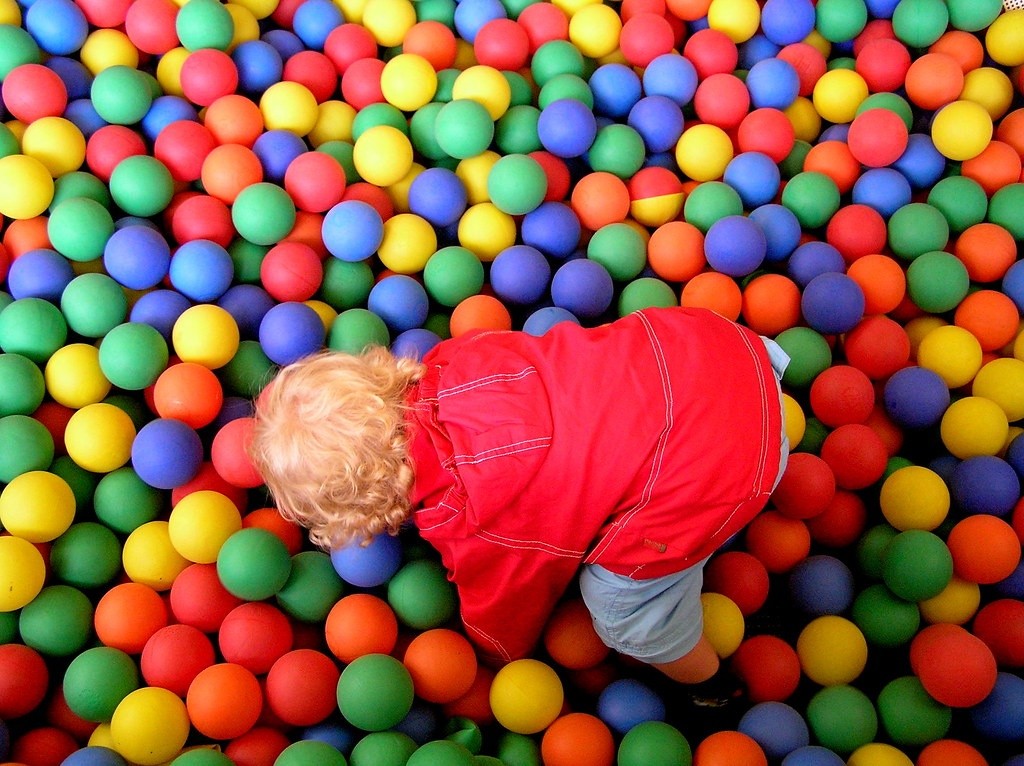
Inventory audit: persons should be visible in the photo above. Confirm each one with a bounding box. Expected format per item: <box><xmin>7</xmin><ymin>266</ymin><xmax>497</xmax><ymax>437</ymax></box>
<box><xmin>248</xmin><ymin>306</ymin><xmax>790</xmax><ymax>758</ymax></box>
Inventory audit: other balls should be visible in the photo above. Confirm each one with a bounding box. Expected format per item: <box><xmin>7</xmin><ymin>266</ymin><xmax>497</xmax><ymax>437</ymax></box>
<box><xmin>536</xmin><ymin>97</ymin><xmax>599</xmax><ymax>159</ymax></box>
<box><xmin>130</xmin><ymin>417</ymin><xmax>204</xmax><ymax>490</ymax></box>
<box><xmin>336</xmin><ymin>645</ymin><xmax>415</xmax><ymax>732</ymax></box>
<box><xmin>321</xmin><ymin>199</ymin><xmax>384</xmax><ymax>263</ymax></box>
<box><xmin>351</xmin><ymin>123</ymin><xmax>414</xmax><ymax>187</ymax></box>
<box><xmin>103</xmin><ymin>224</ymin><xmax>171</xmax><ymax>291</ymax></box>
<box><xmin>171</xmin><ymin>304</ymin><xmax>240</xmax><ymax>370</ymax></box>
<box><xmin>488</xmin><ymin>658</ymin><xmax>565</xmax><ymax>735</ymax></box>
<box><xmin>1</xmin><ymin>2</ymin><xmax>1024</xmax><ymax>765</ymax></box>
<box><xmin>846</xmin><ymin>107</ymin><xmax>909</xmax><ymax>169</ymax></box>
<box><xmin>186</xmin><ymin>663</ymin><xmax>263</xmax><ymax>740</ymax></box>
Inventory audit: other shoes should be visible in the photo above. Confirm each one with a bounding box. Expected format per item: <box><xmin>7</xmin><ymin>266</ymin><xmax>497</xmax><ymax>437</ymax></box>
<box><xmin>689</xmin><ymin>691</ymin><xmax>735</xmax><ymax>735</ymax></box>
